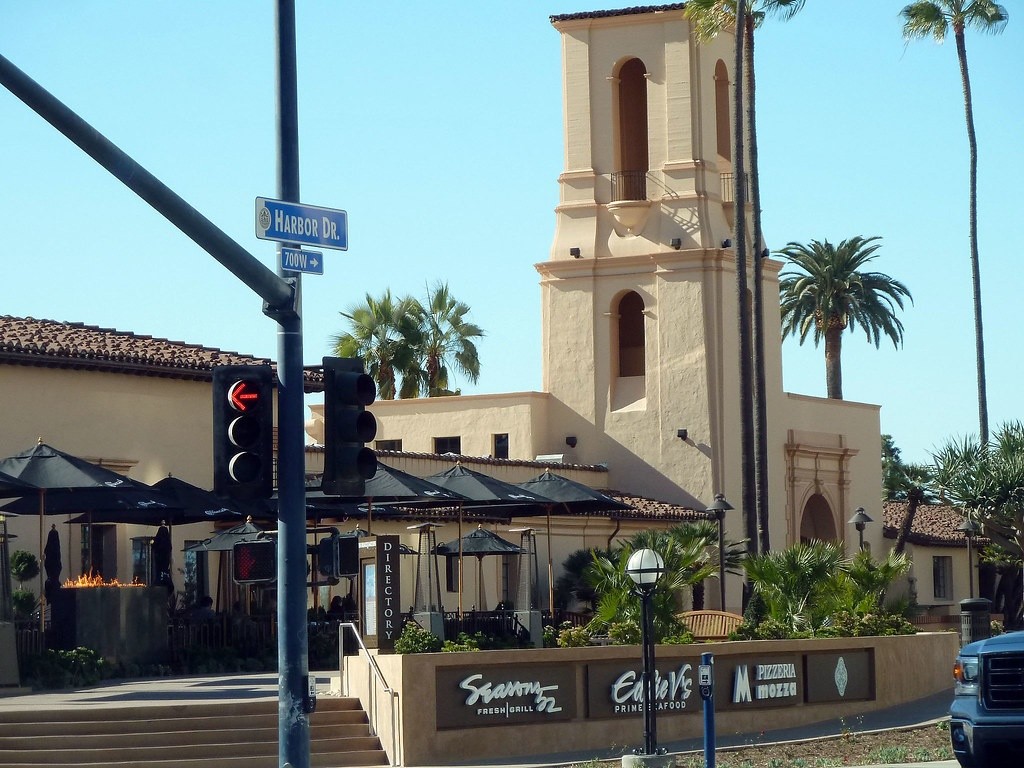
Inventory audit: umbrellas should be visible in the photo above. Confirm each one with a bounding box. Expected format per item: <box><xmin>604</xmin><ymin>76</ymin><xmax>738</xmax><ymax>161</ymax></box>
<box><xmin>0</xmin><ymin>437</ymin><xmax>555</xmax><ymax>637</ymax></box>
<box><xmin>462</xmin><ymin>468</ymin><xmax>637</xmax><ymax>625</ymax></box>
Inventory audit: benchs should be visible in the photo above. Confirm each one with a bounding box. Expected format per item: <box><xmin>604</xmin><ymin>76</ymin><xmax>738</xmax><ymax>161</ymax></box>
<box><xmin>670</xmin><ymin>610</ymin><xmax>744</xmax><ymax>642</ymax></box>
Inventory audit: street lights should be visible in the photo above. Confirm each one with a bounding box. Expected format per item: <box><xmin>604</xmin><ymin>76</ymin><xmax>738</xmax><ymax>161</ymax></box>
<box><xmin>705</xmin><ymin>492</ymin><xmax>736</xmax><ymax>613</ymax></box>
<box><xmin>953</xmin><ymin>518</ymin><xmax>982</xmax><ymax>599</ymax></box>
<box><xmin>847</xmin><ymin>507</ymin><xmax>876</xmax><ymax>556</ymax></box>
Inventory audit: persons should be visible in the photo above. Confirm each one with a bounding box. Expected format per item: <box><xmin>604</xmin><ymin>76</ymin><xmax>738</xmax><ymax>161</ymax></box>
<box><xmin>193</xmin><ymin>596</ymin><xmax>215</xmax><ymax>625</ymax></box>
<box><xmin>330</xmin><ymin>595</ymin><xmax>342</xmax><ymax>614</ymax></box>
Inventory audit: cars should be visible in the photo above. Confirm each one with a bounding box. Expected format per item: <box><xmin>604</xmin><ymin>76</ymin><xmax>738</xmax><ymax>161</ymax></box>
<box><xmin>949</xmin><ymin>629</ymin><xmax>1024</xmax><ymax>768</ymax></box>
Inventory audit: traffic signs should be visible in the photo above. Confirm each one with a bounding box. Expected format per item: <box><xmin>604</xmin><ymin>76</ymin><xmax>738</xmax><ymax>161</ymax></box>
<box><xmin>254</xmin><ymin>196</ymin><xmax>349</xmax><ymax>252</ymax></box>
<box><xmin>280</xmin><ymin>246</ymin><xmax>325</xmax><ymax>276</ymax></box>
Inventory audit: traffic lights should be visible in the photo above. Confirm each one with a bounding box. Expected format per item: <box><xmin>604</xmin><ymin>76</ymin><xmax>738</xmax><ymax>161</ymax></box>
<box><xmin>231</xmin><ymin>536</ymin><xmax>278</xmax><ymax>585</ymax></box>
<box><xmin>320</xmin><ymin>355</ymin><xmax>377</xmax><ymax>496</ymax></box>
<box><xmin>211</xmin><ymin>364</ymin><xmax>274</xmax><ymax>500</ymax></box>
<box><xmin>317</xmin><ymin>532</ymin><xmax>359</xmax><ymax>578</ymax></box>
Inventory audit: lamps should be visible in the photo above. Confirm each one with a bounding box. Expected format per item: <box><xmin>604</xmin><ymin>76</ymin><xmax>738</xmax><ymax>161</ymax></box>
<box><xmin>722</xmin><ymin>239</ymin><xmax>732</xmax><ymax>248</ymax></box>
<box><xmin>677</xmin><ymin>429</ymin><xmax>687</xmax><ymax>440</ymax></box>
<box><xmin>570</xmin><ymin>248</ymin><xmax>580</xmax><ymax>259</ymax></box>
<box><xmin>670</xmin><ymin>238</ymin><xmax>682</xmax><ymax>250</ymax></box>
<box><xmin>565</xmin><ymin>437</ymin><xmax>577</xmax><ymax>448</ymax></box>
<box><xmin>761</xmin><ymin>248</ymin><xmax>770</xmax><ymax>259</ymax></box>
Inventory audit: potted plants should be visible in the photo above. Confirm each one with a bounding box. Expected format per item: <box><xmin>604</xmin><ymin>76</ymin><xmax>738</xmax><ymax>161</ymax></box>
<box><xmin>20</xmin><ymin>647</ymin><xmax>107</xmax><ymax>691</ymax></box>
